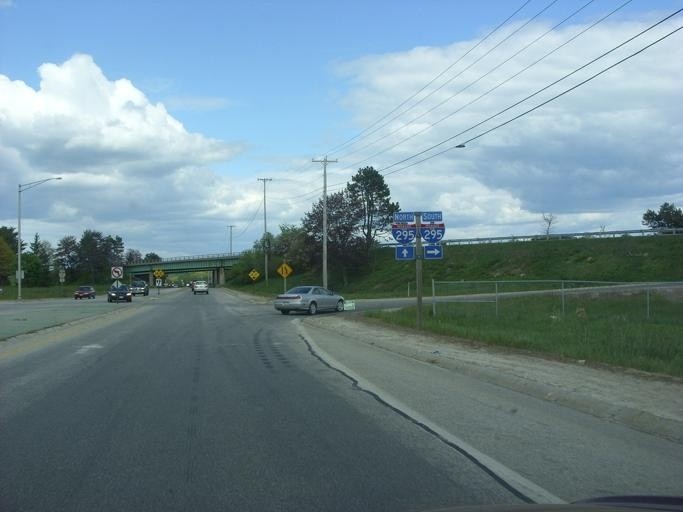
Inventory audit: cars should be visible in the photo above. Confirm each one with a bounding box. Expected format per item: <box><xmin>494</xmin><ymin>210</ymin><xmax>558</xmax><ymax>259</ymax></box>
<box><xmin>75</xmin><ymin>286</ymin><xmax>96</xmax><ymax>299</ymax></box>
<box><xmin>275</xmin><ymin>286</ymin><xmax>345</xmax><ymax>315</ymax></box>
<box><xmin>130</xmin><ymin>281</ymin><xmax>150</xmax><ymax>296</ymax></box>
<box><xmin>108</xmin><ymin>283</ymin><xmax>131</xmax><ymax>302</ymax></box>
<box><xmin>169</xmin><ymin>279</ymin><xmax>196</xmax><ymax>291</ymax></box>
<box><xmin>193</xmin><ymin>280</ymin><xmax>208</xmax><ymax>294</ymax></box>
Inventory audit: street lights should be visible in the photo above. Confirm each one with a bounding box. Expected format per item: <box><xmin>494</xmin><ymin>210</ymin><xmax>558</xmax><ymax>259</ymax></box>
<box><xmin>16</xmin><ymin>176</ymin><xmax>62</xmax><ymax>300</ymax></box>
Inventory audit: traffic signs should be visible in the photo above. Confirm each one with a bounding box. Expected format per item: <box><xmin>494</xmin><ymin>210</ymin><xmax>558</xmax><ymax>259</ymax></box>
<box><xmin>420</xmin><ymin>212</ymin><xmax>441</xmax><ymax>222</ymax></box>
<box><xmin>419</xmin><ymin>223</ymin><xmax>445</xmax><ymax>244</ymax></box>
<box><xmin>393</xmin><ymin>213</ymin><xmax>413</xmax><ymax>222</ymax></box>
<box><xmin>391</xmin><ymin>224</ymin><xmax>415</xmax><ymax>244</ymax></box>
